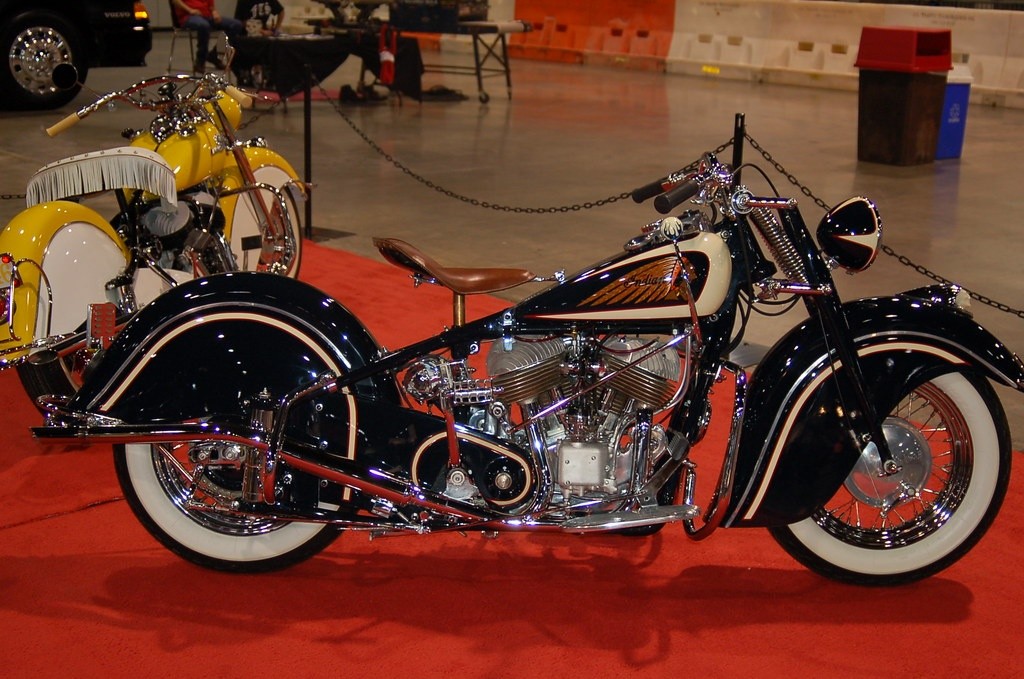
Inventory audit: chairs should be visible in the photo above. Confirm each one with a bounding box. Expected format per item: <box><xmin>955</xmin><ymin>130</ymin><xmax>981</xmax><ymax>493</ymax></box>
<box><xmin>168</xmin><ymin>0</ymin><xmax>219</xmax><ymax>75</ymax></box>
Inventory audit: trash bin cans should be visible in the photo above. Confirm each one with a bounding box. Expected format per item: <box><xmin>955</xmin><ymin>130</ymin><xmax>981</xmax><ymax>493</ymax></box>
<box><xmin>852</xmin><ymin>25</ymin><xmax>955</xmax><ymax>165</ymax></box>
<box><xmin>933</xmin><ymin>62</ymin><xmax>974</xmax><ymax>160</ymax></box>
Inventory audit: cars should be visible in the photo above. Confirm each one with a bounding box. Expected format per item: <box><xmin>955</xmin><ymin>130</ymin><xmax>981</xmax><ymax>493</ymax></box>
<box><xmin>0</xmin><ymin>0</ymin><xmax>154</xmax><ymax>116</ymax></box>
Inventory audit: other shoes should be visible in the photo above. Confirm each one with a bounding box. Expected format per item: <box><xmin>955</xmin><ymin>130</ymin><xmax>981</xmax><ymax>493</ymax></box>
<box><xmin>193</xmin><ymin>63</ymin><xmax>205</xmax><ymax>74</ymax></box>
<box><xmin>206</xmin><ymin>53</ymin><xmax>226</xmax><ymax>70</ymax></box>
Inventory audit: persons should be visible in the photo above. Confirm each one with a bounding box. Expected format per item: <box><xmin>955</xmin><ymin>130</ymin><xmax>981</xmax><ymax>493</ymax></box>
<box><xmin>168</xmin><ymin>0</ymin><xmax>285</xmax><ymax>73</ymax></box>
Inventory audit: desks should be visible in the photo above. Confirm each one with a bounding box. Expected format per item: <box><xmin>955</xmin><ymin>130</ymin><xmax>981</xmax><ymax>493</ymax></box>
<box><xmin>229</xmin><ymin>33</ymin><xmax>349</xmax><ymax>115</ymax></box>
<box><xmin>305</xmin><ymin>18</ymin><xmax>531</xmax><ymax>103</ymax></box>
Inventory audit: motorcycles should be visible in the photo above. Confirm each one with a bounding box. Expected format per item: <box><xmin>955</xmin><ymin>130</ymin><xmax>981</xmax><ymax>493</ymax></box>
<box><xmin>30</xmin><ymin>146</ymin><xmax>1013</xmax><ymax>589</ymax></box>
<box><xmin>0</xmin><ymin>72</ymin><xmax>312</xmax><ymax>426</ymax></box>
